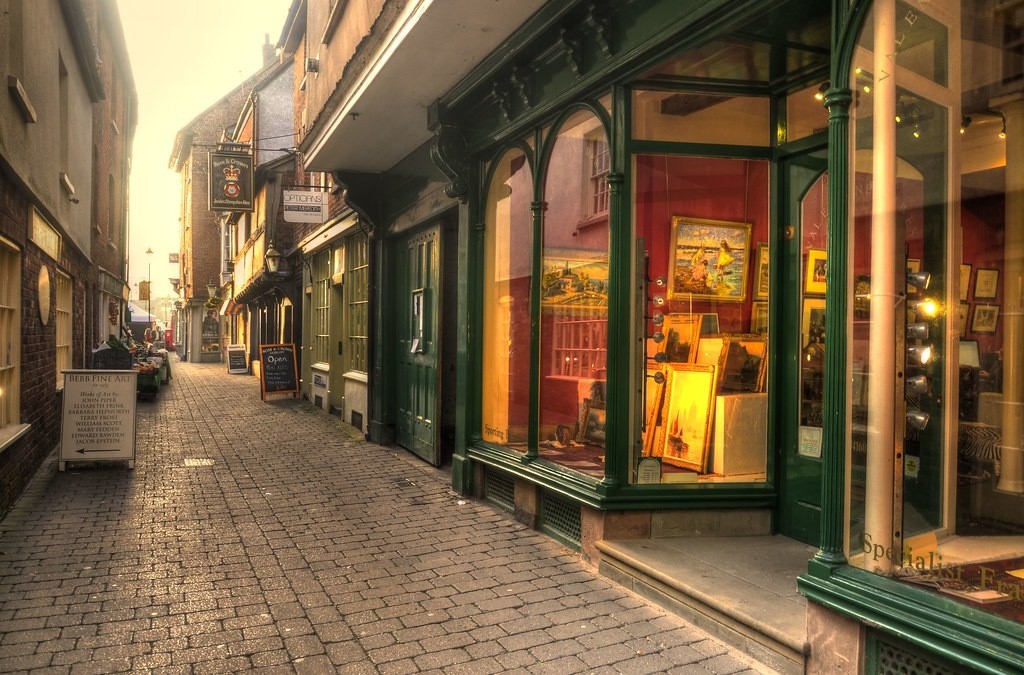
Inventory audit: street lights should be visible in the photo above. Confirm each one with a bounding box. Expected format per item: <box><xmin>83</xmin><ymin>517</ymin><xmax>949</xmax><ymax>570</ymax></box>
<box><xmin>146</xmin><ymin>248</ymin><xmax>155</xmax><ymax>328</ymax></box>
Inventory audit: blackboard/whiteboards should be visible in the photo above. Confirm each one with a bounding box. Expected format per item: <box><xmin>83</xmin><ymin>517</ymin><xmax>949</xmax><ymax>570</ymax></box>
<box><xmin>228</xmin><ymin>350</ymin><xmax>248</xmax><ymax>373</ymax></box>
<box><xmin>259</xmin><ymin>344</ymin><xmax>299</xmax><ymax>395</ymax></box>
<box><xmin>59</xmin><ymin>369</ymin><xmax>141</xmax><ymax>459</ymax></box>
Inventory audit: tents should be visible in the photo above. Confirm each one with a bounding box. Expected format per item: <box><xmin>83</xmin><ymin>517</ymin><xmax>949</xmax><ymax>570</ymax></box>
<box><xmin>129</xmin><ymin>302</ymin><xmax>156</xmax><ymax>329</ymax></box>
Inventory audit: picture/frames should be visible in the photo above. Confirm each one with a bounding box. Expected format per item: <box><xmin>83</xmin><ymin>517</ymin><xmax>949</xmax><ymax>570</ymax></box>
<box><xmin>960</xmin><ymin>263</ymin><xmax>972</xmax><ymax>301</ymax></box>
<box><xmin>960</xmin><ymin>302</ymin><xmax>970</xmax><ymax>338</ymax></box>
<box><xmin>526</xmin><ymin>245</ymin><xmax>609</xmax><ymax>317</ymax></box>
<box><xmin>642</xmin><ymin>362</ymin><xmax>666</xmax><ymax>459</ymax></box>
<box><xmin>657</xmin><ymin>313</ymin><xmax>703</xmax><ymax>363</ymax></box>
<box><xmin>973</xmin><ymin>304</ymin><xmax>999</xmax><ymax>334</ymax></box>
<box><xmin>804</xmin><ymin>247</ymin><xmax>828</xmax><ymax>295</ymax></box>
<box><xmin>973</xmin><ymin>269</ymin><xmax>999</xmax><ymax>299</ymax></box>
<box><xmin>749</xmin><ymin>302</ymin><xmax>768</xmax><ymax>334</ymax></box>
<box><xmin>724</xmin><ymin>332</ymin><xmax>768</xmax><ymax>393</ymax></box>
<box><xmin>701</xmin><ymin>313</ymin><xmax>720</xmax><ymax>336</ymax></box>
<box><xmin>575</xmin><ymin>398</ymin><xmax>606</xmax><ymax>448</ymax></box>
<box><xmin>659</xmin><ymin>363</ymin><xmax>720</xmax><ymax>473</ymax></box>
<box><xmin>906</xmin><ymin>259</ymin><xmax>920</xmax><ymax>294</ymax></box>
<box><xmin>801</xmin><ymin>298</ymin><xmax>826</xmax><ymax>350</ymax></box>
<box><xmin>754</xmin><ymin>242</ymin><xmax>768</xmax><ymax>301</ymax></box>
<box><xmin>666</xmin><ymin>217</ymin><xmax>751</xmax><ymax>302</ymax></box>
<box><xmin>696</xmin><ymin>332</ymin><xmax>731</xmax><ymax>395</ymax></box>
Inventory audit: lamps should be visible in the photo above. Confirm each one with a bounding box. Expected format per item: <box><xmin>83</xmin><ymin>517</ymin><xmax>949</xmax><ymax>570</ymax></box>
<box><xmin>907</xmin><ymin>375</ymin><xmax>927</xmax><ymax>392</ymax></box>
<box><xmin>908</xmin><ymin>346</ymin><xmax>930</xmax><ymax>364</ymax></box>
<box><xmin>896</xmin><ymin>105</ymin><xmax>914</xmax><ymax>123</ymax></box>
<box><xmin>908</xmin><ymin>323</ymin><xmax>929</xmax><ymax>339</ymax></box>
<box><xmin>815</xmin><ymin>82</ymin><xmax>831</xmax><ymax>101</ymax></box>
<box><xmin>913</xmin><ymin>121</ymin><xmax>930</xmax><ymax>138</ymax></box>
<box><xmin>960</xmin><ymin>115</ymin><xmax>971</xmax><ymax>134</ymax></box>
<box><xmin>991</xmin><ymin>110</ymin><xmax>1006</xmax><ymax>138</ymax></box>
<box><xmin>908</xmin><ymin>411</ymin><xmax>929</xmax><ymax>430</ymax></box>
<box><xmin>908</xmin><ymin>272</ymin><xmax>930</xmax><ymax>290</ymax></box>
<box><xmin>265</xmin><ymin>240</ymin><xmax>301</xmax><ymax>273</ymax></box>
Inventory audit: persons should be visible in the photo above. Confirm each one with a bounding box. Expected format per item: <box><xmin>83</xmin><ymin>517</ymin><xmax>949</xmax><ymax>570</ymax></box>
<box><xmin>152</xmin><ymin>326</ymin><xmax>160</xmax><ymax>341</ymax></box>
<box><xmin>203</xmin><ymin>311</ymin><xmax>219</xmax><ymax>333</ymax></box>
<box><xmin>144</xmin><ymin>328</ymin><xmax>153</xmax><ymax>344</ymax></box>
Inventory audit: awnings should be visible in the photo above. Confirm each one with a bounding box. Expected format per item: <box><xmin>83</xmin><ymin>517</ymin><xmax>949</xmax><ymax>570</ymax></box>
<box><xmin>220</xmin><ymin>299</ymin><xmax>246</xmax><ymax>315</ymax></box>
